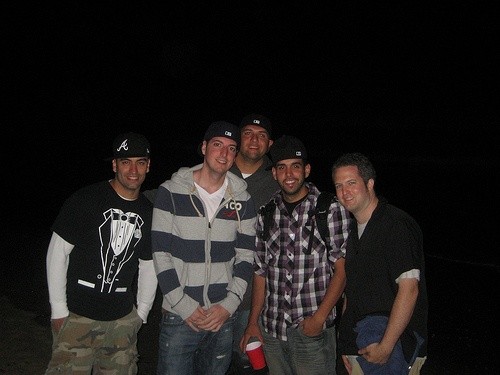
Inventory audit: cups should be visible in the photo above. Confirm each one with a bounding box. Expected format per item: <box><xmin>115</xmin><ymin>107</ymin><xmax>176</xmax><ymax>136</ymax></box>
<box><xmin>245</xmin><ymin>341</ymin><xmax>266</xmax><ymax>370</ymax></box>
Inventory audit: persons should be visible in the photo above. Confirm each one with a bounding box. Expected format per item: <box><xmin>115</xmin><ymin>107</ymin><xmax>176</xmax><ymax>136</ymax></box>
<box><xmin>331</xmin><ymin>153</ymin><xmax>427</xmax><ymax>375</ymax></box>
<box><xmin>240</xmin><ymin>136</ymin><xmax>351</xmax><ymax>375</ymax></box>
<box><xmin>142</xmin><ymin>113</ymin><xmax>282</xmax><ymax>375</ymax></box>
<box><xmin>44</xmin><ymin>131</ymin><xmax>159</xmax><ymax>375</ymax></box>
<box><xmin>151</xmin><ymin>121</ymin><xmax>257</xmax><ymax>375</ymax></box>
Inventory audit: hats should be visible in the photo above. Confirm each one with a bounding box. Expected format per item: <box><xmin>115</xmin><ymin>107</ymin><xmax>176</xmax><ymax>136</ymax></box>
<box><xmin>103</xmin><ymin>132</ymin><xmax>151</xmax><ymax>165</ymax></box>
<box><xmin>240</xmin><ymin>112</ymin><xmax>273</xmax><ymax>136</ymax></box>
<box><xmin>204</xmin><ymin>121</ymin><xmax>240</xmax><ymax>146</ymax></box>
<box><xmin>265</xmin><ymin>135</ymin><xmax>306</xmax><ymax>172</ymax></box>
